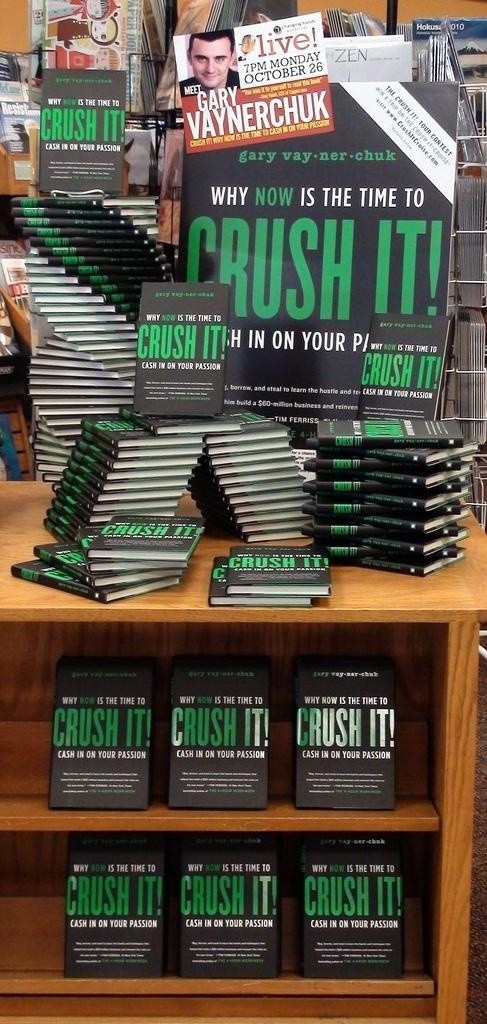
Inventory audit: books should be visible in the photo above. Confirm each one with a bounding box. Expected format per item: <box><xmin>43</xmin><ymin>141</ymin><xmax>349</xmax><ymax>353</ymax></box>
<box><xmin>129</xmin><ymin>51</ymin><xmax>157</xmax><ymax>115</ymax></box>
<box><xmin>122</xmin><ymin>407</ymin><xmax>248</xmax><ymax>436</ymax></box>
<box><xmin>449</xmin><ymin>319</ymin><xmax>485</xmax><ymax>446</ymax></box>
<box><xmin>200</xmin><ymin>414</ymin><xmax>311</xmax><ymax>544</ymax></box>
<box><xmin>177</xmin><ymin>841</ymin><xmax>282</xmax><ymax>980</ymax></box>
<box><xmin>28</xmin><ymin>409</ymin><xmax>85</xmax><ymax>483</ymax></box>
<box><xmin>133</xmin><ymin>282</ymin><xmax>230</xmax><ymax>417</ymax></box>
<box><xmin>207</xmin><ymin>543</ymin><xmax>335</xmax><ymax>610</ymax></box>
<box><xmin>326</xmin><ymin>8</ymin><xmax>390</xmax><ymax>38</ymax></box>
<box><xmin>298</xmin><ymin>836</ymin><xmax>404</xmax><ymax>979</ymax></box>
<box><xmin>11</xmin><ymin>187</ymin><xmax>178</xmax><ymax>318</ymax></box>
<box><xmin>415</xmin><ymin>24</ymin><xmax>484</xmax><ymax>171</ymax></box>
<box><xmin>294</xmin><ymin>653</ymin><xmax>397</xmax><ymax>813</ymax></box>
<box><xmin>61</xmin><ymin>846</ymin><xmax>168</xmax><ymax>979</ymax></box>
<box><xmin>49</xmin><ymin>654</ymin><xmax>154</xmax><ymax>811</ymax></box>
<box><xmin>53</xmin><ymin>50</ymin><xmax>127</xmax><ymax>65</ymax></box>
<box><xmin>178</xmin><ymin>81</ymin><xmax>453</xmax><ymax>421</ymax></box>
<box><xmin>7</xmin><ymin>517</ymin><xmax>204</xmax><ymax>601</ymax></box>
<box><xmin>22</xmin><ymin>50</ymin><xmax>40</xmax><ymax>103</ymax></box>
<box><xmin>24</xmin><ymin>320</ymin><xmax>137</xmax><ymax>405</ymax></box>
<box><xmin>451</xmin><ymin>174</ymin><xmax>485</xmax><ymax>311</ymax></box>
<box><xmin>167</xmin><ymin>653</ymin><xmax>269</xmax><ymax>810</ymax></box>
<box><xmin>299</xmin><ymin>417</ymin><xmax>479</xmax><ymax>579</ymax></box>
<box><xmin>37</xmin><ymin>68</ymin><xmax>128</xmax><ymax>196</ymax></box>
<box><xmin>45</xmin><ymin>418</ymin><xmax>203</xmax><ymax>543</ymax></box>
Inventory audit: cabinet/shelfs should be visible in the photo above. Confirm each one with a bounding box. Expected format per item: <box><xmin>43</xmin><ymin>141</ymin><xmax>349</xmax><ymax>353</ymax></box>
<box><xmin>0</xmin><ymin>479</ymin><xmax>487</xmax><ymax>1024</ymax></box>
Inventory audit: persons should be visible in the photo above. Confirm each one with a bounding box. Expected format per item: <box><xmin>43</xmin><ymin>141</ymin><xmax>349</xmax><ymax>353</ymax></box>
<box><xmin>178</xmin><ymin>29</ymin><xmax>241</xmax><ymax>102</ymax></box>
